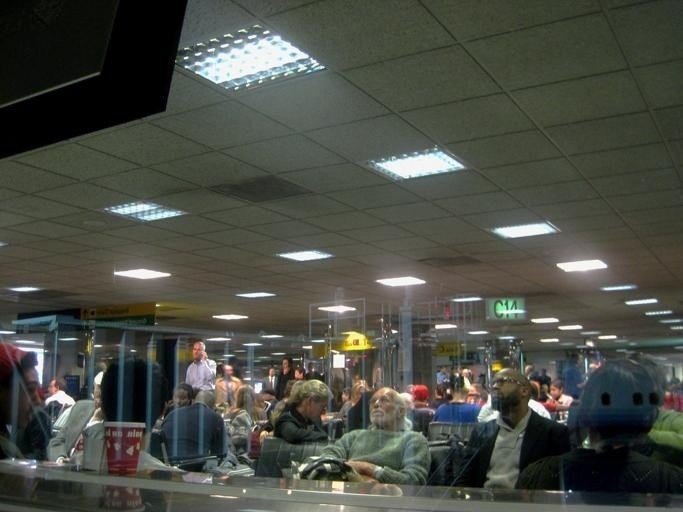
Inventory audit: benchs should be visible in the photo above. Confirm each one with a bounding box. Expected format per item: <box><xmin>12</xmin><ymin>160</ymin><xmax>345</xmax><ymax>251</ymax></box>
<box><xmin>254</xmin><ymin>421</ymin><xmax>476</xmax><ymax>487</ymax></box>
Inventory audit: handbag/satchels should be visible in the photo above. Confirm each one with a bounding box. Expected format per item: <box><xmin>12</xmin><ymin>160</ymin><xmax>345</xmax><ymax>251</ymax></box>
<box><xmin>248</xmin><ymin>426</ymin><xmax>268</xmax><ymax>458</ymax></box>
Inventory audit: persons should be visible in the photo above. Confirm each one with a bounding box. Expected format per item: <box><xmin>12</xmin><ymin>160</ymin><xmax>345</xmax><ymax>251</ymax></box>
<box><xmin>2</xmin><ymin>338</ymin><xmax>683</xmax><ymax>476</ymax></box>
<box><xmin>320</xmin><ymin>383</ymin><xmax>430</xmax><ymax>487</ymax></box>
<box><xmin>514</xmin><ymin>356</ymin><xmax>683</xmax><ymax>495</ymax></box>
<box><xmin>451</xmin><ymin>365</ymin><xmax>572</xmax><ymax>489</ymax></box>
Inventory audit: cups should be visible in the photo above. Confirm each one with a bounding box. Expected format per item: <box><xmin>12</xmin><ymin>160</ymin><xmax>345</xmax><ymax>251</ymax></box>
<box><xmin>103</xmin><ymin>421</ymin><xmax>147</xmax><ymax>477</ymax></box>
<box><xmin>98</xmin><ymin>482</ymin><xmax>146</xmax><ymax>512</ymax></box>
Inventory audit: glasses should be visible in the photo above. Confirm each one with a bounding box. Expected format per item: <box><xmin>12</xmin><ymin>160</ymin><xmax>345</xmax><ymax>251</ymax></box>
<box><xmin>485</xmin><ymin>375</ymin><xmax>523</xmax><ymax>392</ymax></box>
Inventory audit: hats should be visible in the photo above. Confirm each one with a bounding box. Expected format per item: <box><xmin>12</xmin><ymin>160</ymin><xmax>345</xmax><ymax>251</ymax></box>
<box><xmin>415</xmin><ymin>385</ymin><xmax>431</xmax><ymax>399</ymax></box>
